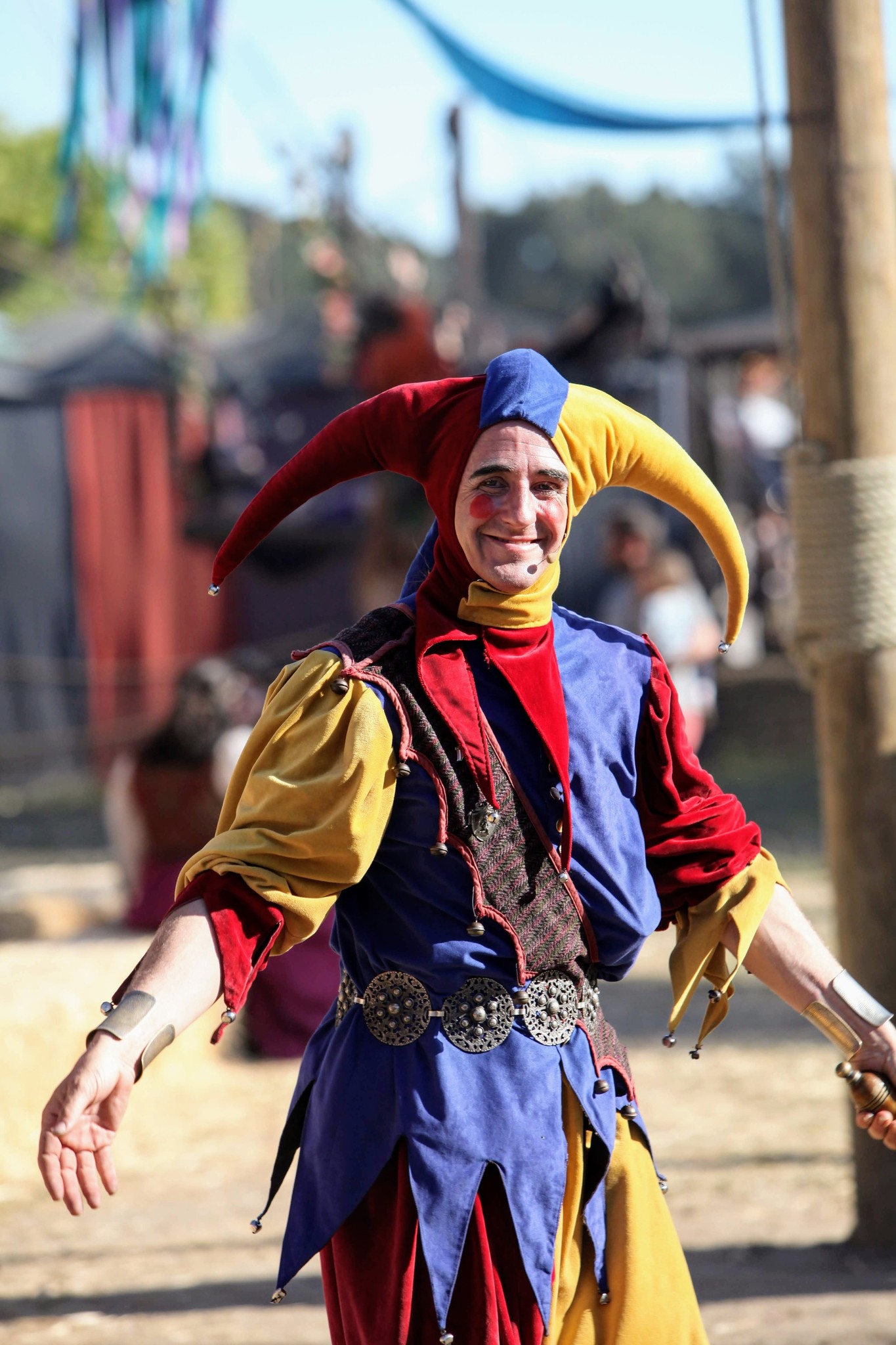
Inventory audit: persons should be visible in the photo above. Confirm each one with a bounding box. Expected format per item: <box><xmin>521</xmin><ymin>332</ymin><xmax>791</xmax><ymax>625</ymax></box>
<box><xmin>733</xmin><ymin>345</ymin><xmax>802</xmax><ymax>513</ymax></box>
<box><xmin>37</xmin><ymin>347</ymin><xmax>896</xmax><ymax>1345</ymax></box>
<box><xmin>593</xmin><ymin>498</ymin><xmax>722</xmax><ymax>761</ymax></box>
<box><xmin>103</xmin><ymin>649</ymin><xmax>346</xmax><ymax>1058</ymax></box>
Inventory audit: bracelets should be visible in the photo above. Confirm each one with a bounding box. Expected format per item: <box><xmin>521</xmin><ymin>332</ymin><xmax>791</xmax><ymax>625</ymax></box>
<box><xmin>799</xmin><ymin>966</ymin><xmax>895</xmax><ymax>1063</ymax></box>
<box><xmin>85</xmin><ymin>991</ymin><xmax>176</xmax><ymax>1086</ymax></box>
